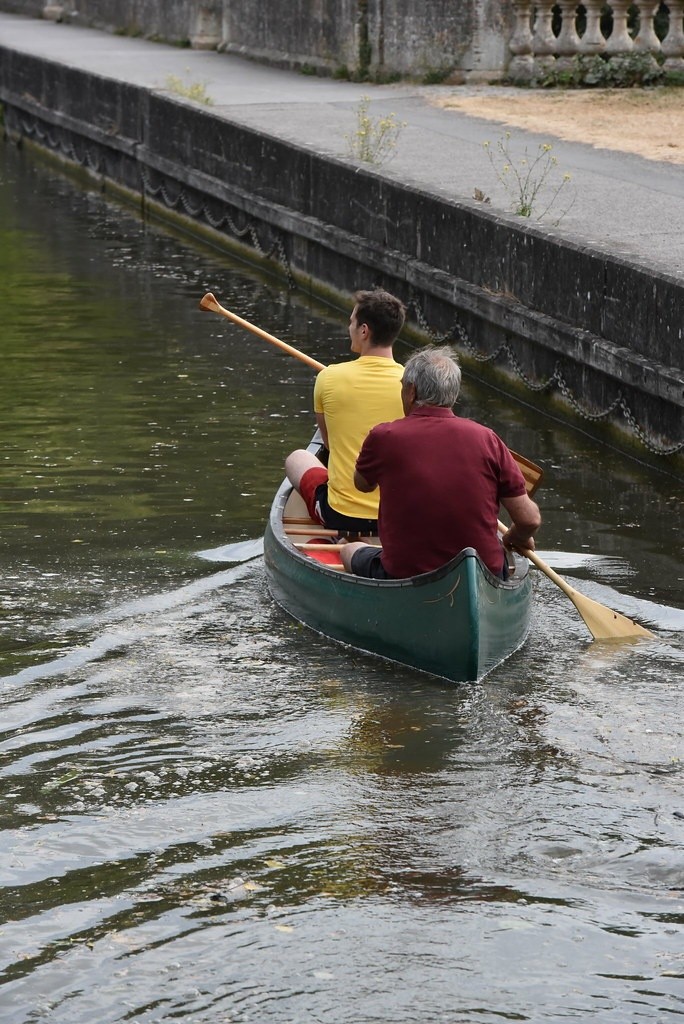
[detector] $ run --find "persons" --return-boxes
[340,343,541,580]
[284,288,408,538]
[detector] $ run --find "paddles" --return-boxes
[198,291,545,500]
[497,517,658,639]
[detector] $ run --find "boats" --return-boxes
[264,426,533,687]
